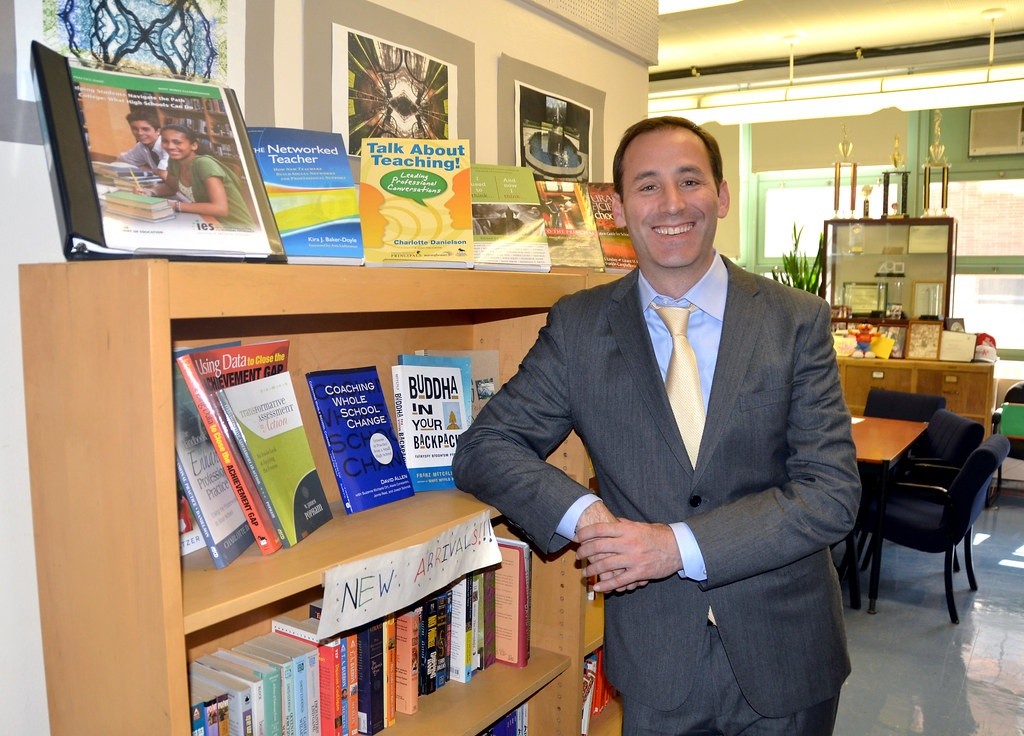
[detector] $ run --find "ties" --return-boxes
[649,302,706,470]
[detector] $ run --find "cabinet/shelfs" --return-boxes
[835,356,993,449]
[20,260,631,736]
[823,217,955,328]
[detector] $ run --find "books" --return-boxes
[469,164,552,273]
[244,126,366,266]
[305,365,415,514]
[103,189,177,223]
[90,159,164,189]
[162,338,333,570]
[580,648,618,736]
[187,537,535,735]
[584,181,639,274]
[390,349,500,494]
[359,135,474,270]
[535,180,606,273]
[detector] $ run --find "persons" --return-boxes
[116,108,254,232]
[836,305,847,318]
[847,322,886,356]
[887,303,900,318]
[451,116,862,736]
[341,689,348,699]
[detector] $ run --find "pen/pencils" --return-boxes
[130,169,142,190]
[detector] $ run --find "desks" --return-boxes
[844,412,926,613]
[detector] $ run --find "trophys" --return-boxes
[879,134,911,219]
[831,120,860,222]
[921,110,953,218]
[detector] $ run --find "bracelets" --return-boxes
[176,200,182,212]
[145,188,158,197]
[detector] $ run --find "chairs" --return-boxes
[835,385,1010,624]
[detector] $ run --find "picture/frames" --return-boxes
[876,322,909,359]
[905,319,945,360]
